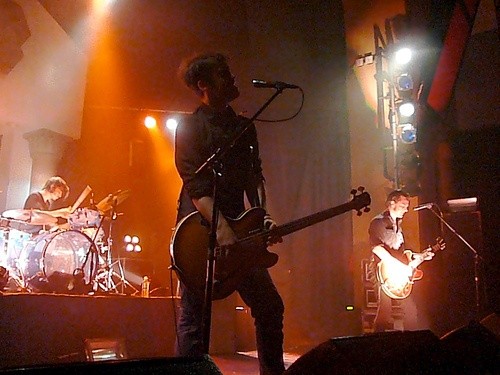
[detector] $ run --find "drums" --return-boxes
[17,229,99,294]
[1,226,32,273]
[70,207,105,241]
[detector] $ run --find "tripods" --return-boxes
[96,217,141,296]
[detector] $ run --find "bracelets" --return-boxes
[264,214,271,219]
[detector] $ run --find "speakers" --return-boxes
[1,354,224,375]
[277,313,500,374]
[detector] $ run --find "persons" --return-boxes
[24,176,71,229]
[368,190,435,333]
[174,48,286,375]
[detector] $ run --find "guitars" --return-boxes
[169,184,373,300]
[376,237,446,300]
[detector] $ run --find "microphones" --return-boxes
[414,203,433,211]
[252,79,300,89]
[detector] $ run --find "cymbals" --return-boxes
[2,209,57,224]
[96,189,130,211]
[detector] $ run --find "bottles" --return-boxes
[142,276,150,298]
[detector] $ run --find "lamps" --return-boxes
[396,72,413,98]
[399,122,417,144]
